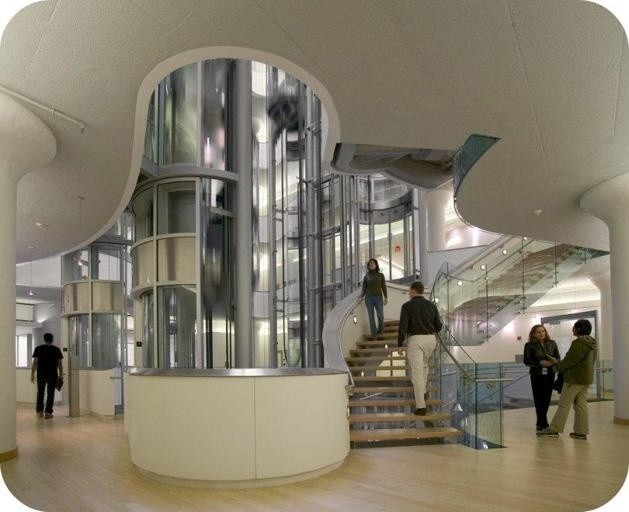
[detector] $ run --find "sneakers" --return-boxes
[538,428,559,437]
[536,426,543,434]
[38,412,43,417]
[45,412,53,419]
[570,432,586,440]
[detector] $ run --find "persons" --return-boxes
[536,319,598,441]
[358,258,388,342]
[30,333,64,420]
[397,281,443,417]
[523,324,561,435]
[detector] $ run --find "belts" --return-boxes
[409,332,433,336]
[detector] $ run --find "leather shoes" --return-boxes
[379,330,384,334]
[414,409,427,416]
[372,336,378,341]
